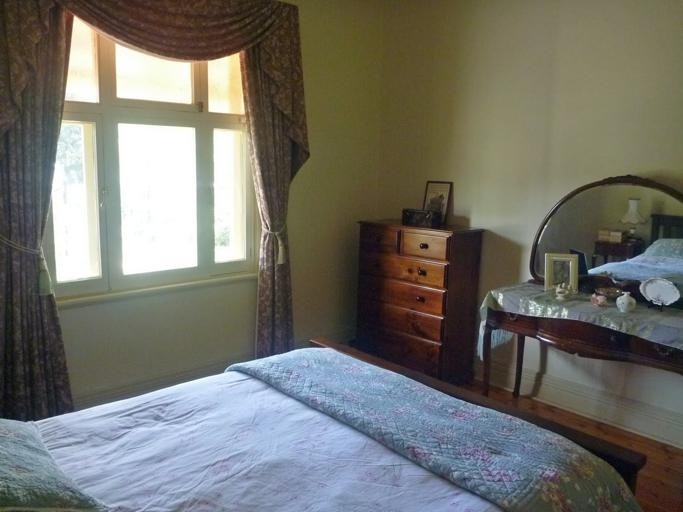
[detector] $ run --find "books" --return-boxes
[597,229,622,243]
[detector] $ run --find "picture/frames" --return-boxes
[543,252,580,295]
[421,178,453,227]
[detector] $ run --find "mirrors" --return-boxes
[528,173,683,310]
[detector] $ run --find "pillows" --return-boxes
[0,419,107,512]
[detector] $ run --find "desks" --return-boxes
[589,240,643,266]
[481,281,683,416]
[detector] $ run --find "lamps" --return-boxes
[620,194,647,244]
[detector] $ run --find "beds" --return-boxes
[0,333,650,512]
[589,213,683,302]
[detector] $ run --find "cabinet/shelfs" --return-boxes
[357,216,483,383]
